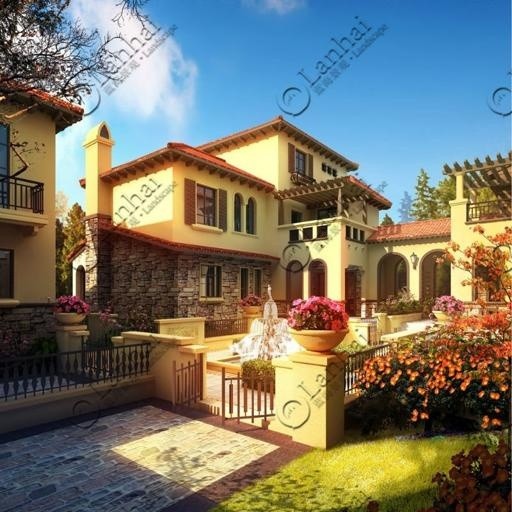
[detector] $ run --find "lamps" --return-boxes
[410,252,417,269]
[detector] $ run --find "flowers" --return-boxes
[287,296,349,330]
[429,295,465,319]
[240,294,264,307]
[52,295,90,314]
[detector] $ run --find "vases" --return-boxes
[54,313,87,324]
[243,306,262,313]
[432,311,453,322]
[288,327,349,352]
